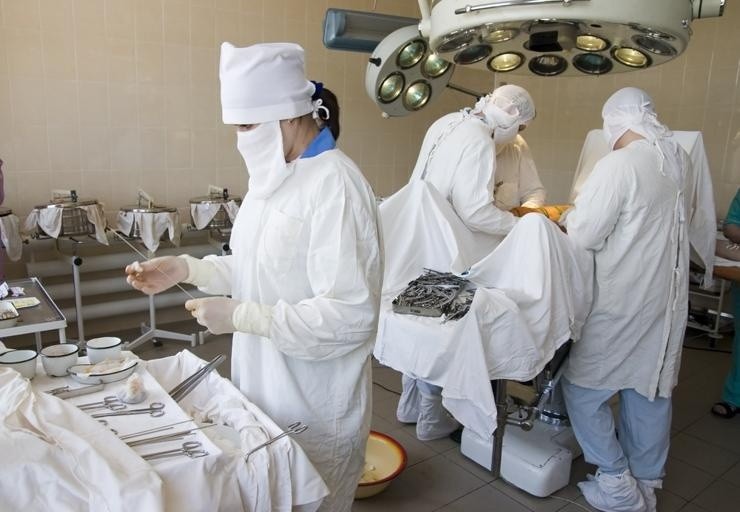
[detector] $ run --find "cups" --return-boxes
[0,348,38,380]
[38,344,80,377]
[84,336,122,365]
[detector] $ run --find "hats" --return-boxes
[600,87,655,120]
[218,41,330,124]
[492,85,536,123]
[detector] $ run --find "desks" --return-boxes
[0,277,67,352]
[0,350,293,510]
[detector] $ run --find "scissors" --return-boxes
[92,403,165,418]
[76,396,125,412]
[244,422,308,461]
[140,441,208,461]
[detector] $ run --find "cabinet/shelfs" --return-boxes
[686,249,724,333]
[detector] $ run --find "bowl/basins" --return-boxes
[354,430,408,500]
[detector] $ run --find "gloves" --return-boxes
[184,297,273,335]
[125,253,213,296]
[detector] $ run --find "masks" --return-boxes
[602,121,629,150]
[494,124,519,143]
[236,121,292,197]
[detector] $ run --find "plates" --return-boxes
[65,363,139,385]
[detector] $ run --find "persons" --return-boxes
[562,85,694,512]
[125,41,385,510]
[710,185,739,419]
[396,83,543,442]
[494,134,546,211]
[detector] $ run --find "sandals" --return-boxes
[711,402,739,418]
